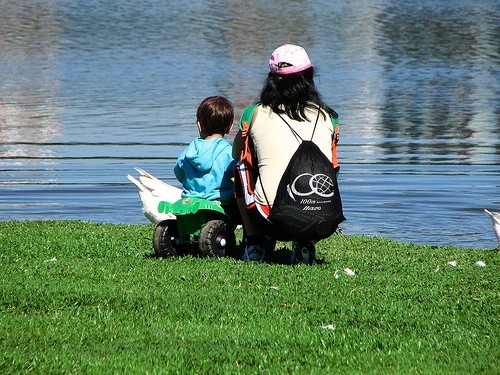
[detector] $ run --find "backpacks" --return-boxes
[258,104,347,241]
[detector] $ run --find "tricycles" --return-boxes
[152,192,277,259]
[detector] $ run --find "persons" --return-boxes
[172,96,246,256]
[233,44,347,262]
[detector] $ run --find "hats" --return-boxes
[269,44,312,74]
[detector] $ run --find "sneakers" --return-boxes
[241,245,265,262]
[291,241,316,266]
